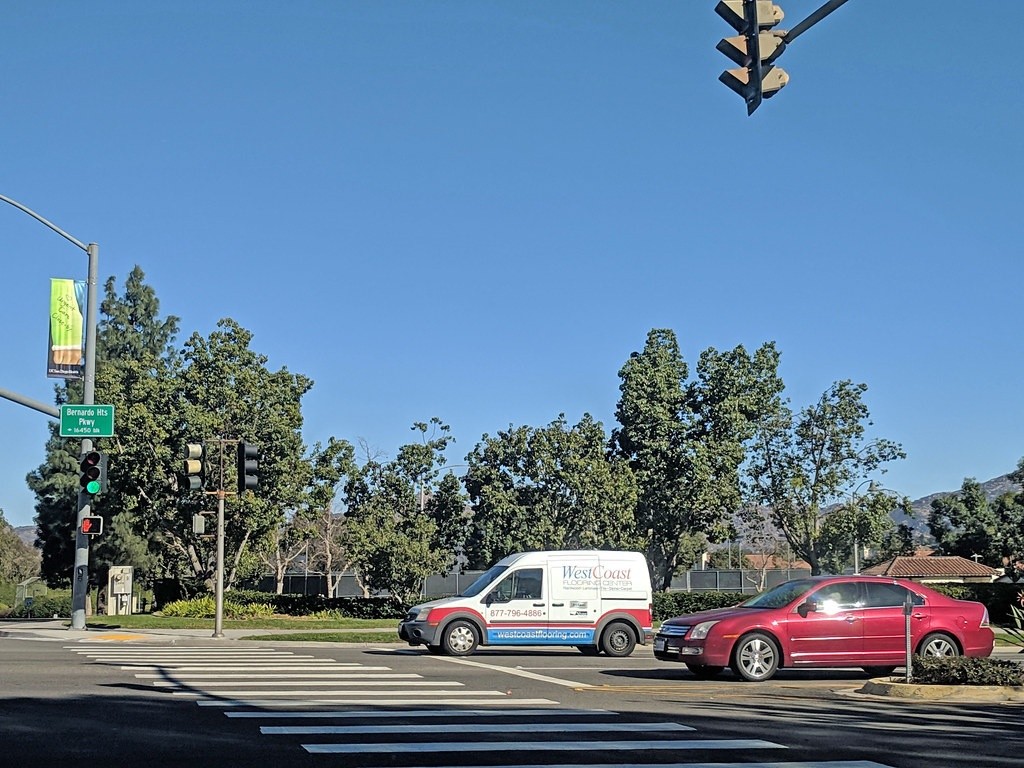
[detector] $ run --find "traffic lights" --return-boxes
[238,443,259,494]
[711,0,789,114]
[182,443,207,491]
[81,451,102,496]
[82,516,104,535]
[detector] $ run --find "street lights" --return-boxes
[852,479,880,574]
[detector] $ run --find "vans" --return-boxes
[397,545,653,657]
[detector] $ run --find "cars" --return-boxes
[653,575,996,683]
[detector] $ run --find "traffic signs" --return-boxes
[60,404,114,436]
